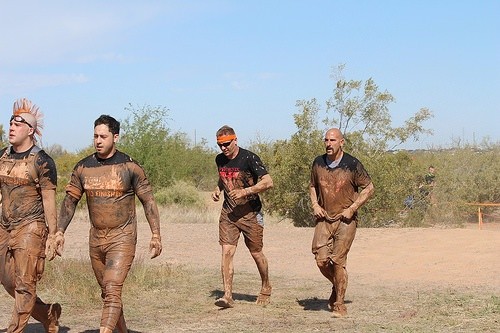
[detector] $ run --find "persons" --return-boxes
[308,128,374,317]
[418,167,440,218]
[56,115,162,333]
[0,98,57,333]
[211,125,275,307]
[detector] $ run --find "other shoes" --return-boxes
[329,286,336,305]
[43,303,61,333]
[214,296,234,308]
[256,284,272,307]
[333,302,349,318]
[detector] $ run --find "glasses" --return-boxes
[217,139,233,147]
[10,115,33,128]
